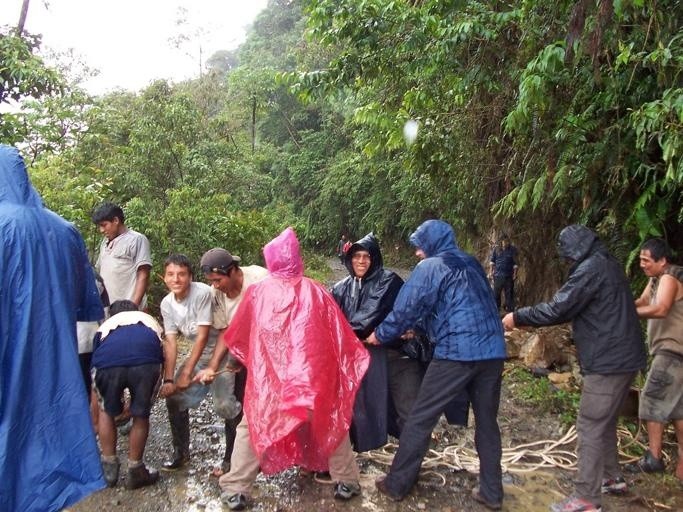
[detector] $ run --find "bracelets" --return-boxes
[163,378,175,384]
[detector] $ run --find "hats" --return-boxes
[200,248,242,274]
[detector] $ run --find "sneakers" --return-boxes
[376,474,403,500]
[125,464,158,490]
[314,471,335,482]
[100,461,119,488]
[601,477,627,494]
[551,495,601,511]
[472,488,503,508]
[213,460,230,476]
[625,451,666,473]
[334,483,361,499]
[220,493,246,510]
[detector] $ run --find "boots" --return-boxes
[162,396,190,471]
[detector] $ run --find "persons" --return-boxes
[217,226,371,512]
[624,237,683,476]
[191,247,322,478]
[0,145,108,511]
[365,218,505,510]
[501,223,648,512]
[90,204,155,434]
[335,235,347,266]
[487,232,519,315]
[314,231,426,485]
[87,299,163,490]
[72,268,109,405]
[159,254,241,474]
